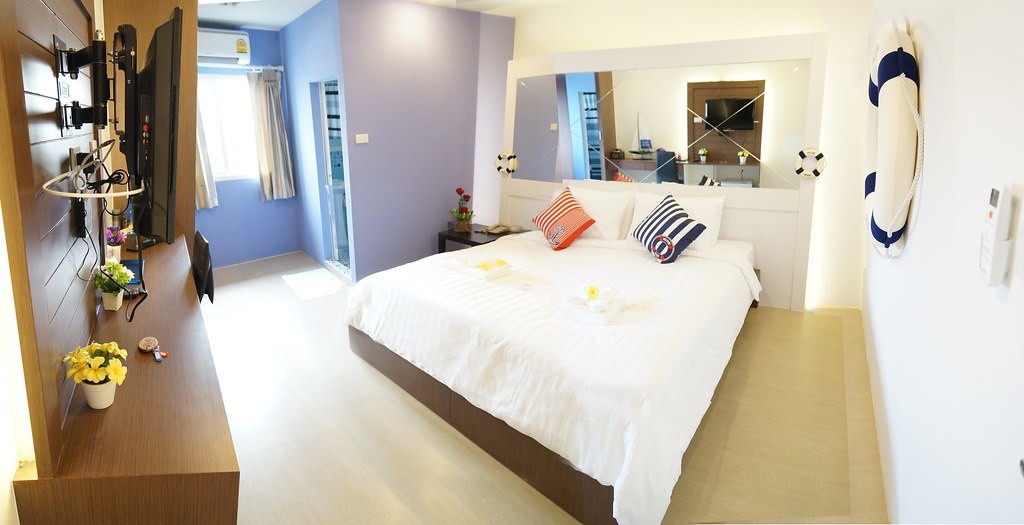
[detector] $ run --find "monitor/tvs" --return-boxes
[705,98,754,131]
[112,5,183,244]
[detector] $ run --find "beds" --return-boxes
[347,230,761,525]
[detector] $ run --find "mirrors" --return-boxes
[510,58,811,190]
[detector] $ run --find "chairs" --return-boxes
[655,151,680,181]
[180,231,218,300]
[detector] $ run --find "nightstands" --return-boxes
[438,222,511,255]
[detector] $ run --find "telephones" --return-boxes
[978,183,1013,287]
[486,224,509,234]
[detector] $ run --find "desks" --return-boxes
[607,159,684,172]
[682,162,761,185]
[54,229,241,524]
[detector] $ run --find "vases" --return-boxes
[454,220,472,232]
[99,288,124,311]
[700,155,706,162]
[81,376,120,409]
[740,156,747,163]
[101,243,121,263]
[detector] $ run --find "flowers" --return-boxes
[738,149,748,157]
[91,261,134,292]
[448,188,476,220]
[698,148,707,155]
[61,340,128,384]
[103,227,130,243]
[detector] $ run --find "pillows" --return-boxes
[625,194,727,246]
[630,192,707,264]
[612,173,633,183]
[698,174,725,186]
[532,190,594,251]
[548,188,638,240]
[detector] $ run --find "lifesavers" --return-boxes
[863,21,919,260]
[495,152,516,174]
[794,149,825,178]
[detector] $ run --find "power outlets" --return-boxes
[740,168,745,173]
[89,140,99,159]
[70,146,82,171]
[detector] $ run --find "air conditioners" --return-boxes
[191,30,251,66]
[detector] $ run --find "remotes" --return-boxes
[122,288,139,299]
